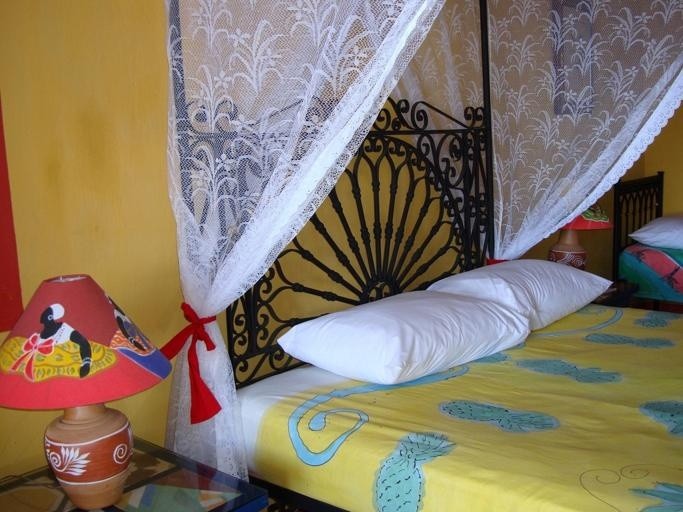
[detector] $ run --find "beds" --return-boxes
[184,102,683,510]
[609,171,683,303]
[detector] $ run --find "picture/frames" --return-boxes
[550,2,594,117]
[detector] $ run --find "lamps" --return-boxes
[0,263,173,508]
[546,178,612,273]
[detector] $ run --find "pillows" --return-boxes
[427,249,613,332]
[628,212,683,250]
[276,250,531,386]
